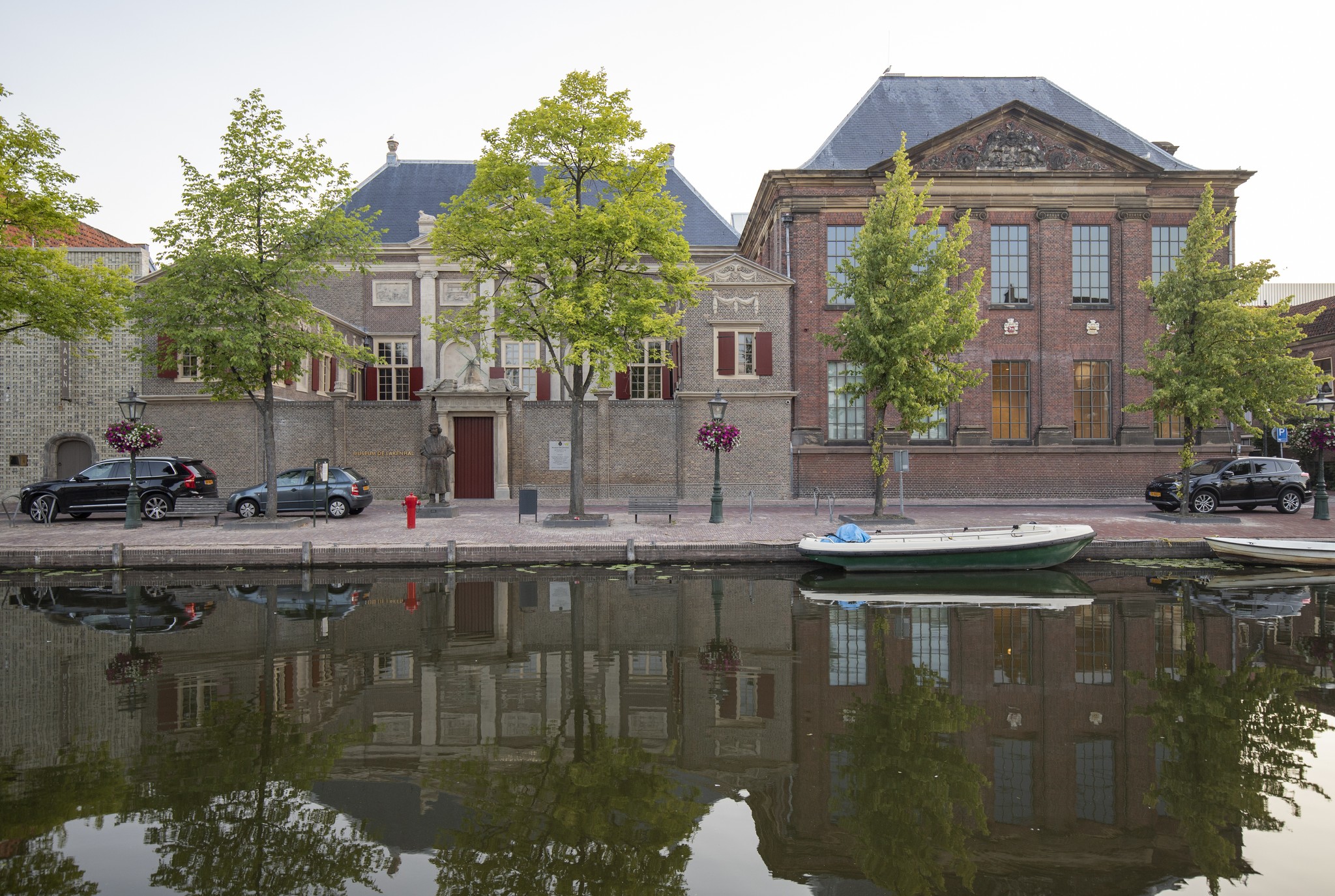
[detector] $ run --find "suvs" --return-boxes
[8,586,220,635]
[1144,457,1313,514]
[19,457,219,522]
[1144,576,1313,620]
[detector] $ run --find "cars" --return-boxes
[225,467,373,522]
[225,582,374,620]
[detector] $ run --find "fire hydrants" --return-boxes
[404,582,420,615]
[401,492,420,528]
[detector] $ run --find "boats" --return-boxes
[796,521,1097,573]
[1205,563,1334,590]
[796,567,1097,612]
[1203,537,1335,567]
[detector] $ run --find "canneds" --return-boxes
[574,517,579,520]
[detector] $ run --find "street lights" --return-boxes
[706,387,728,524]
[1305,388,1335,520]
[1257,405,1274,457]
[116,386,149,530]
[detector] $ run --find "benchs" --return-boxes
[163,496,229,527]
[627,495,680,523]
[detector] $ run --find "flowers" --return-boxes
[103,421,164,457]
[694,421,743,453]
[697,638,744,676]
